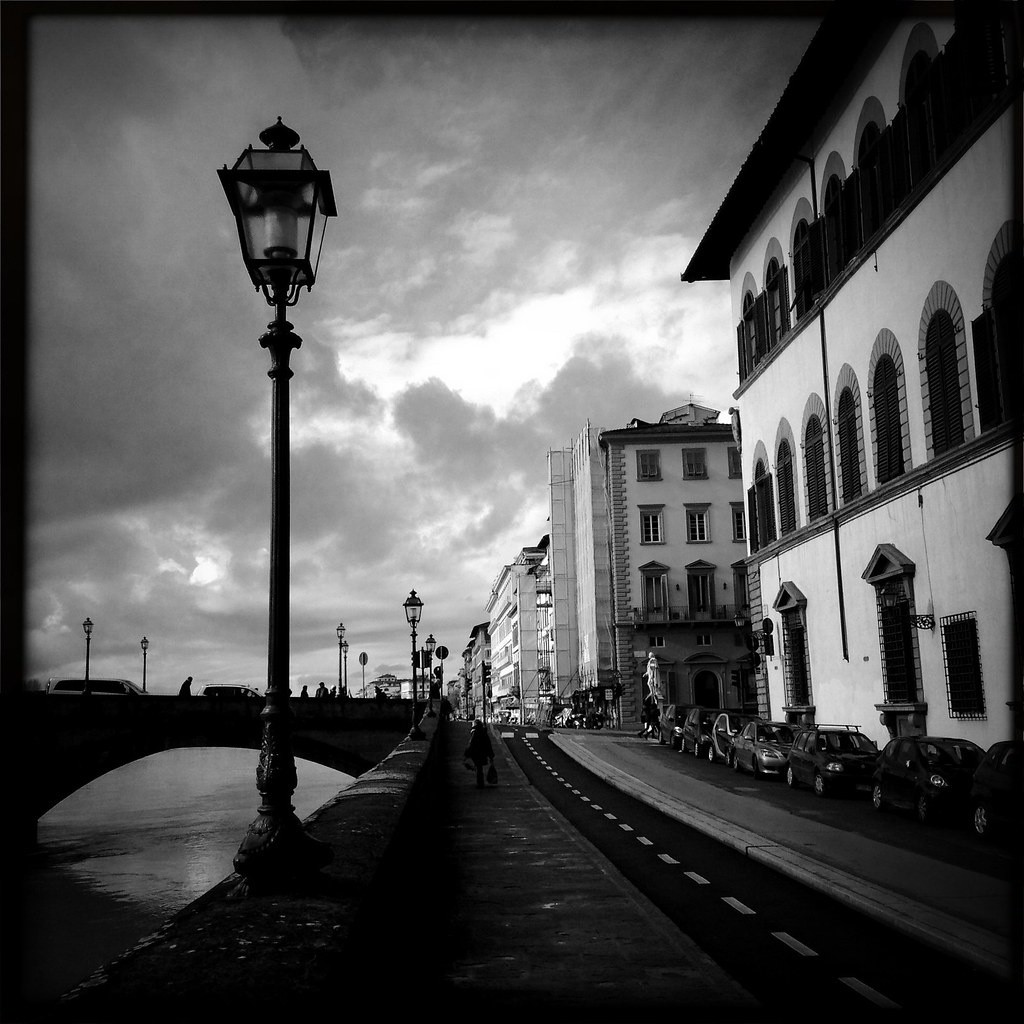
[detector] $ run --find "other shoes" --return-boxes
[476,786,484,789]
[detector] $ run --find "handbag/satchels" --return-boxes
[486,765,498,784]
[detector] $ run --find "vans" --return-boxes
[46,674,151,697]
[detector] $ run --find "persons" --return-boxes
[637,700,658,738]
[300,685,308,697]
[315,682,328,698]
[463,719,495,789]
[179,676,193,695]
[375,686,387,699]
[642,704,661,740]
[643,651,664,701]
[427,674,440,699]
[329,685,337,697]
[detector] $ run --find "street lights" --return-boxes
[81,618,93,698]
[216,116,336,870]
[139,637,150,693]
[426,634,437,718]
[402,590,426,740]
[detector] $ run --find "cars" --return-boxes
[873,735,987,821]
[708,712,762,767]
[969,739,1012,841]
[196,684,266,697]
[658,704,699,747]
[786,724,882,797]
[682,707,737,759]
[733,719,795,777]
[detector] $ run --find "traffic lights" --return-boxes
[464,675,472,693]
[481,663,491,683]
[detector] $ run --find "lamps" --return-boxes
[878,583,935,629]
[733,610,765,640]
